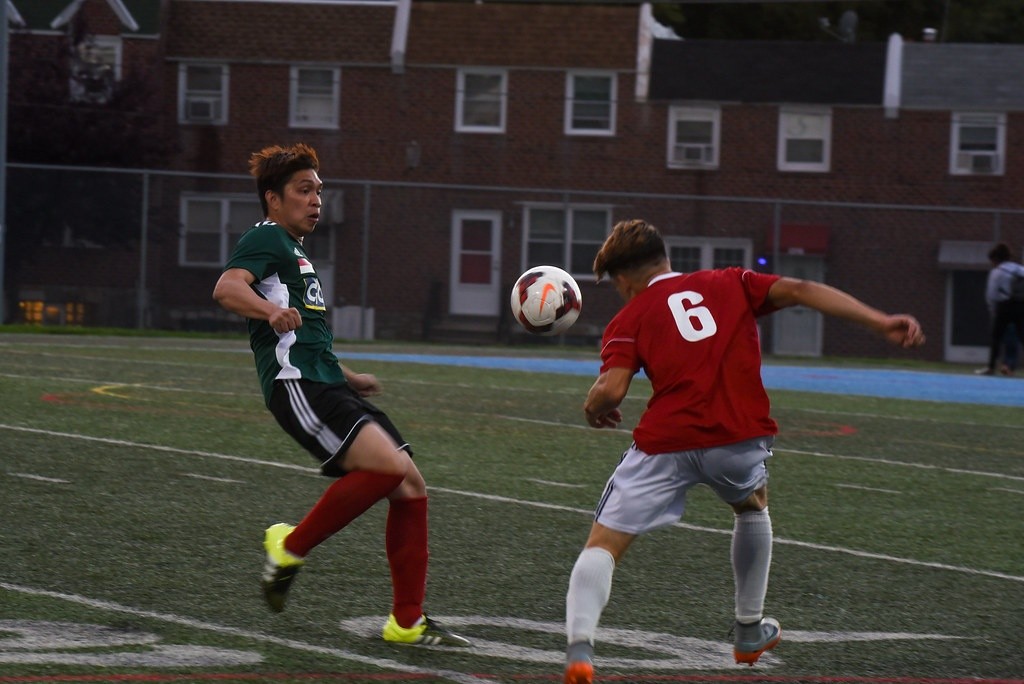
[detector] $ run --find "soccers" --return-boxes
[510,265,583,335]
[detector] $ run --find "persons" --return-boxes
[564,218,924,684]
[212,142,472,648]
[975,244,1024,375]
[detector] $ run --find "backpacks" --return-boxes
[996,267,1024,314]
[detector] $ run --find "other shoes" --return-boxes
[998,364,1015,377]
[975,367,994,375]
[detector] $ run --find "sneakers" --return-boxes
[382,612,471,649]
[566,641,595,684]
[261,523,305,611]
[735,617,781,666]
